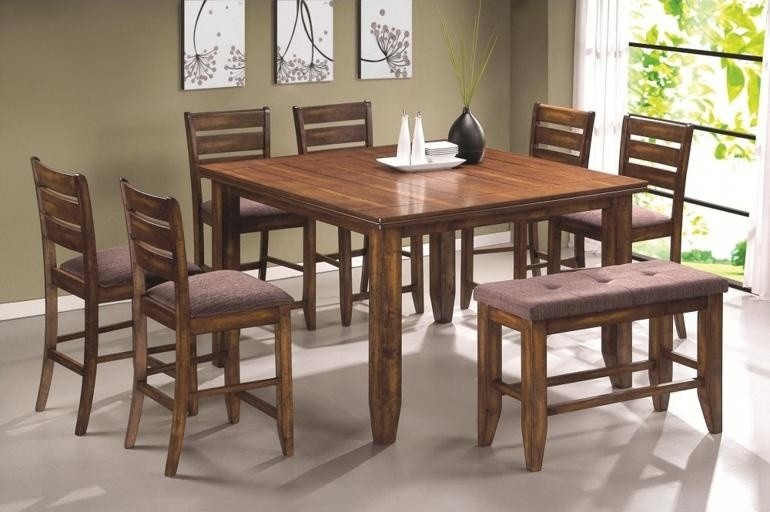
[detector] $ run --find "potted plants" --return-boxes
[438,1,499,169]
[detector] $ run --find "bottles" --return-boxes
[411,112,425,164]
[396,113,410,165]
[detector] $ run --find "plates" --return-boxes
[376,155,465,172]
[425,141,459,161]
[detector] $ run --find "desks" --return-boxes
[193,136,651,443]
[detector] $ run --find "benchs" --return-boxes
[473,257,736,472]
